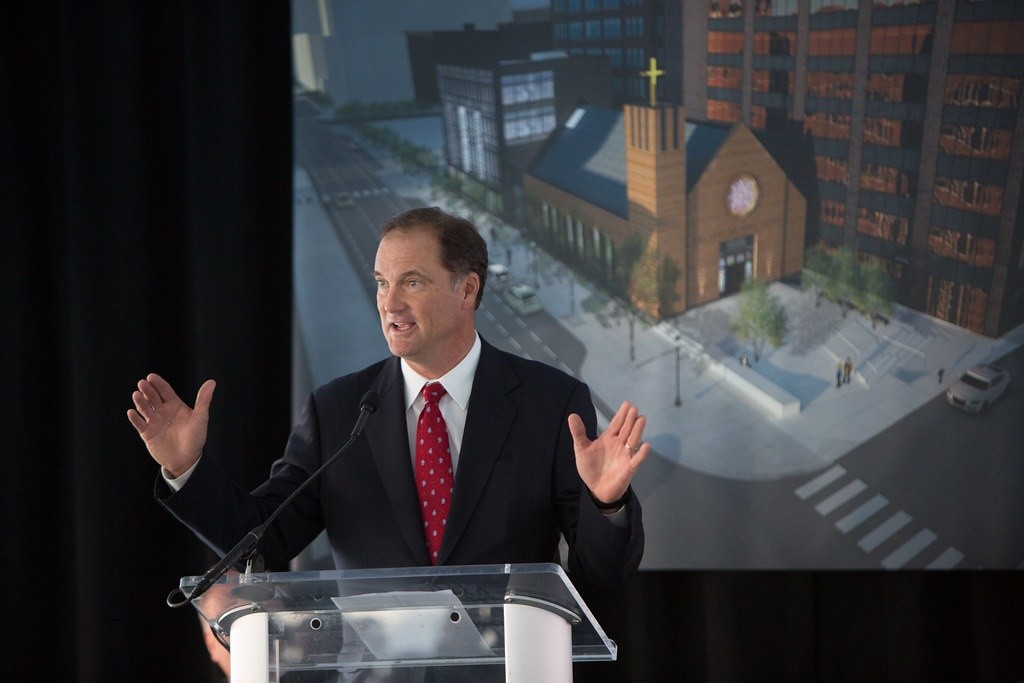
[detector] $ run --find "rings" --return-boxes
[625,442,638,451]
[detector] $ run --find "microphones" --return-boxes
[349,391,381,437]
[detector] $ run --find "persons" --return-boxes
[127,207,652,683]
[836,360,853,388]
[939,369,945,383]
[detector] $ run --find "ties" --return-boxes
[417,382,455,563]
